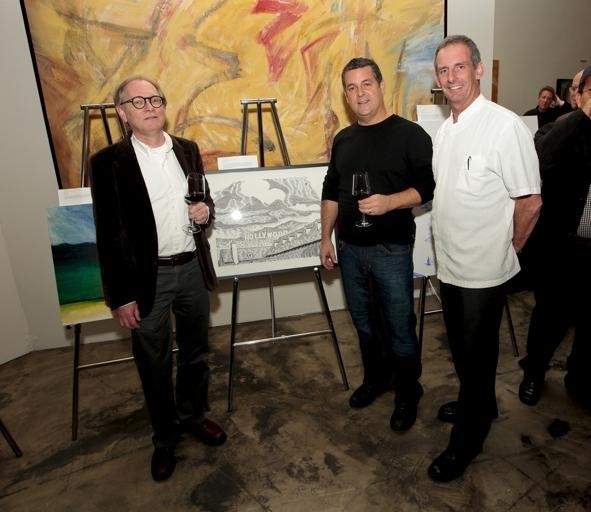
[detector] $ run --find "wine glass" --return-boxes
[352,170,374,229]
[181,171,207,236]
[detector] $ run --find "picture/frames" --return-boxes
[21,0,448,190]
[202,162,339,280]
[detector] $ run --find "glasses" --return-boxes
[118,95,166,110]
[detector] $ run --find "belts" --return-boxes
[156,251,200,268]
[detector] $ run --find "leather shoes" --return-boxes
[429,444,482,483]
[438,401,500,423]
[390,380,423,431]
[177,414,228,446]
[150,430,179,483]
[349,381,393,409]
[519,370,546,406]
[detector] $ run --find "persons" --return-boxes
[318,56,435,434]
[91,77,228,482]
[517,66,591,408]
[424,34,544,484]
